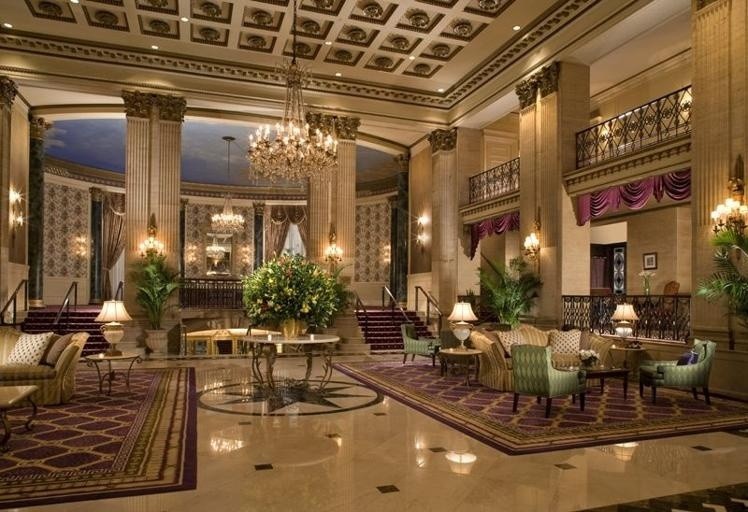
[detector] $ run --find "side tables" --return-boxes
[440,349,485,390]
[84,353,141,395]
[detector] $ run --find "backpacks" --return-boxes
[676,350,698,366]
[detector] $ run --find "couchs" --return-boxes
[473,327,594,392]
[0,325,90,407]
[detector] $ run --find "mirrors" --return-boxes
[205,233,233,277]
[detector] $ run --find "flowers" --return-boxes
[578,349,600,361]
[241,252,342,328]
[637,270,657,280]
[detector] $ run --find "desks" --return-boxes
[242,335,343,405]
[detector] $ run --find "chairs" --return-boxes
[510,342,588,418]
[638,339,717,405]
[400,323,439,368]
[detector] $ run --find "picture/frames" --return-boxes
[643,252,657,270]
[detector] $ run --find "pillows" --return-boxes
[7,330,54,365]
[497,330,526,357]
[45,333,73,365]
[551,328,582,353]
[677,352,698,365]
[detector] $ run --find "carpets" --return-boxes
[332,351,748,457]
[0,366,197,509]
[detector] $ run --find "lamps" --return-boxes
[206,237,226,268]
[448,298,479,352]
[710,197,746,240]
[141,239,166,257]
[524,232,541,262]
[94,301,134,357]
[609,305,640,347]
[246,0,338,183]
[207,134,246,235]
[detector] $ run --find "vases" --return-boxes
[585,359,596,368]
[280,317,302,338]
[644,280,651,297]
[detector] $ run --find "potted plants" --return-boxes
[129,249,185,353]
